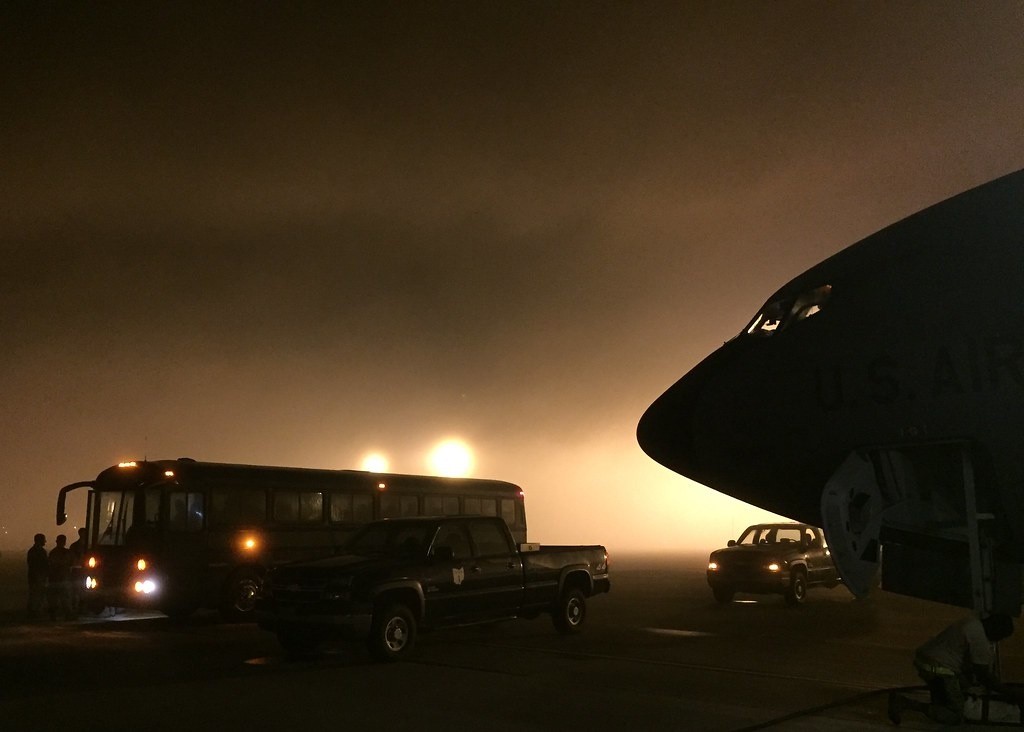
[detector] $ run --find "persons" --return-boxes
[26,528,87,619]
[888,614,1014,727]
[174,498,187,524]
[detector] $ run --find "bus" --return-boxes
[54,457,528,620]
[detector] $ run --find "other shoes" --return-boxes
[888,691,904,725]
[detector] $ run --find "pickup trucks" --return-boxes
[706,521,844,606]
[242,514,612,660]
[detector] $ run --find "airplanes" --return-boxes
[638,167,1024,623]
